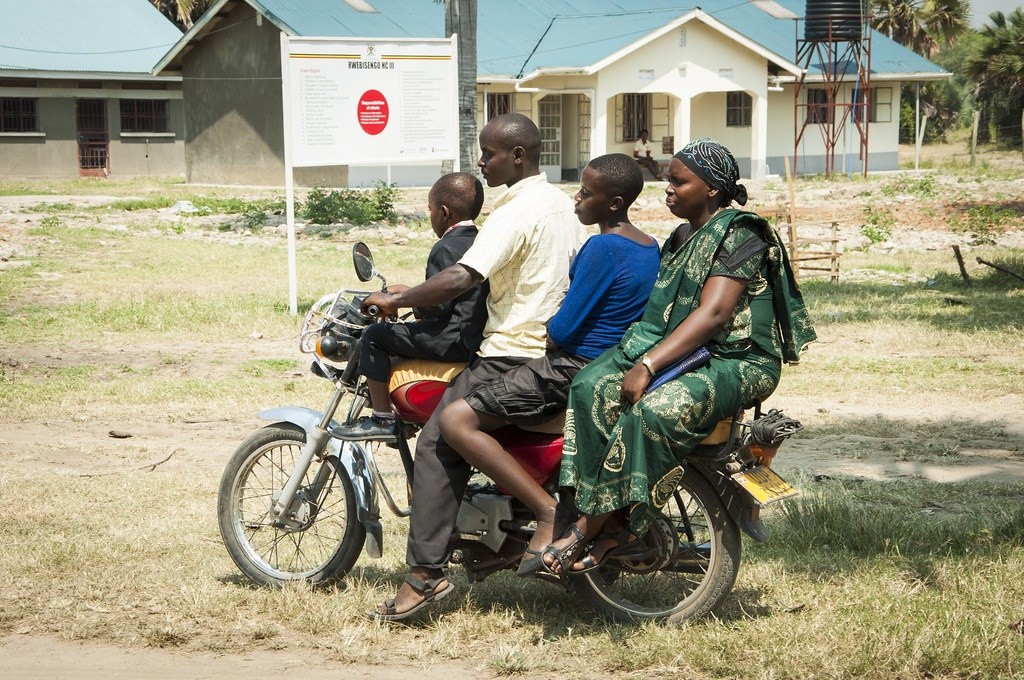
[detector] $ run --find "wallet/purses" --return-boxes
[643,345,711,395]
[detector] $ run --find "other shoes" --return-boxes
[517,507,573,577]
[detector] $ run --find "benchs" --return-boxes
[640,162,668,174]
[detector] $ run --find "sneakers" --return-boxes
[330,416,400,443]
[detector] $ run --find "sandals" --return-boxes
[568,527,641,575]
[368,568,454,620]
[540,513,602,576]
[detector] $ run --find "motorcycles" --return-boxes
[218,242,804,629]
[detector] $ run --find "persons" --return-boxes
[436,155,661,577]
[540,137,819,581]
[327,172,490,440]
[631,128,668,182]
[358,113,592,622]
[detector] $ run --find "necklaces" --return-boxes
[682,227,692,245]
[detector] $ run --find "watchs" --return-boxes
[641,353,656,377]
